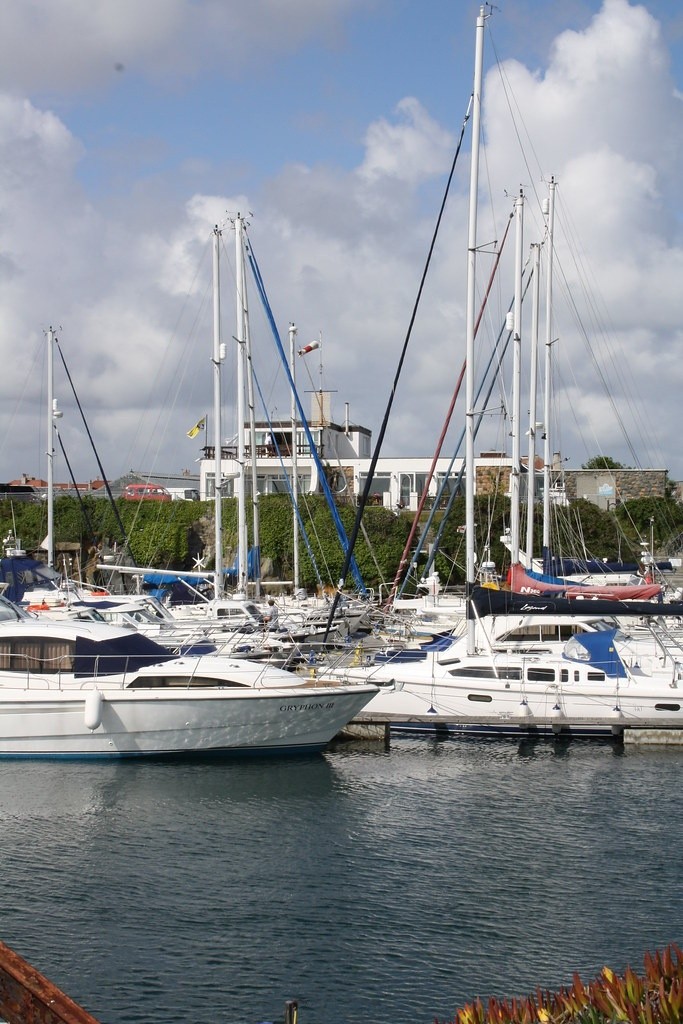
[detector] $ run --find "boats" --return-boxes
[0,617,381,762]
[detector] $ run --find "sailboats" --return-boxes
[295,1,683,744]
[0,211,370,671]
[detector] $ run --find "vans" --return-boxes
[122,484,171,502]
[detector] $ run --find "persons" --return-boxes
[645,566,655,585]
[262,600,279,633]
[294,583,307,609]
[41,599,50,610]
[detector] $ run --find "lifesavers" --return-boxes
[91,592,109,596]
[27,605,50,610]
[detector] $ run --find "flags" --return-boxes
[187,417,206,439]
